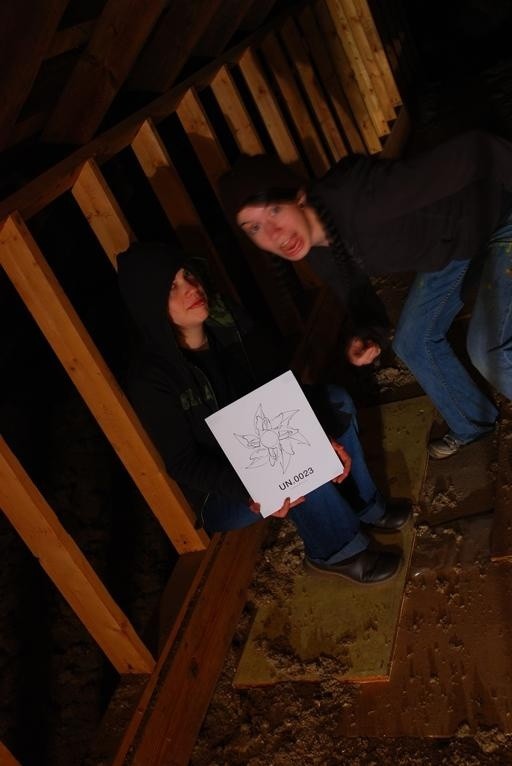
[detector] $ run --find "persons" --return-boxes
[115,246,414,582]
[221,128,512,460]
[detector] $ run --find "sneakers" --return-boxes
[357,508,413,531]
[304,542,401,586]
[429,432,473,460]
